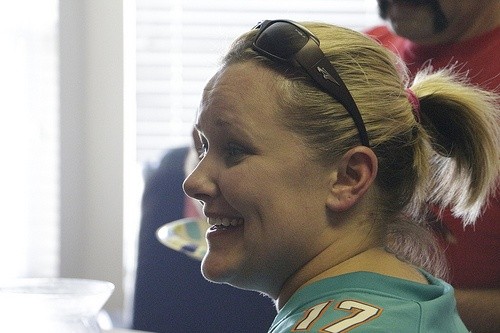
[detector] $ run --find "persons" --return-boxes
[359,0,500,333]
[133,145,278,333]
[183,19,500,333]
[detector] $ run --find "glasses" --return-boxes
[252,18,371,148]
